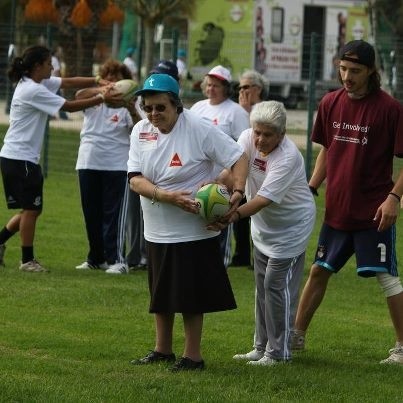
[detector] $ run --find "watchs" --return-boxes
[233,189,245,198]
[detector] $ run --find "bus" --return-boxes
[185,0,370,110]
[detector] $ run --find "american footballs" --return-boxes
[110,79,140,103]
[195,182,233,222]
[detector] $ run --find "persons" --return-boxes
[0,46,270,276]
[126,74,247,373]
[291,40,403,365]
[198,101,315,366]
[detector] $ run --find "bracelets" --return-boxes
[388,192,400,201]
[309,185,318,195]
[151,185,159,205]
[97,93,105,104]
[236,211,240,220]
[94,75,101,84]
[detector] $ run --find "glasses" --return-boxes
[238,84,253,91]
[143,104,171,112]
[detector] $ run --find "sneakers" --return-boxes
[19,259,47,272]
[247,355,285,365]
[105,260,130,274]
[75,261,108,270]
[290,330,305,351]
[232,349,265,360]
[379,342,403,364]
[131,349,175,364]
[0,244,6,265]
[171,357,204,371]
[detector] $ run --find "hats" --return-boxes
[205,65,231,84]
[340,39,375,68]
[149,61,179,82]
[134,74,180,97]
